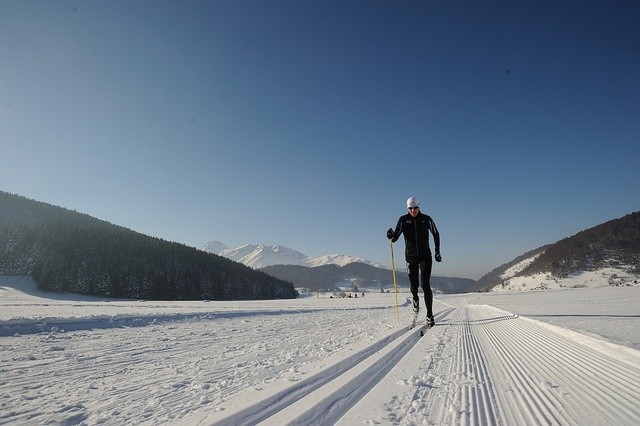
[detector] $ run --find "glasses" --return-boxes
[407,206,419,210]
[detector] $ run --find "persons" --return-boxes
[386,198,442,330]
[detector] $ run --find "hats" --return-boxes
[407,197,420,208]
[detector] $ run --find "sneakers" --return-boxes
[426,315,435,327]
[413,297,419,311]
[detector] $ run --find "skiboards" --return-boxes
[409,323,431,336]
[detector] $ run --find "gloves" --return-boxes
[387,228,394,239]
[435,254,442,262]
